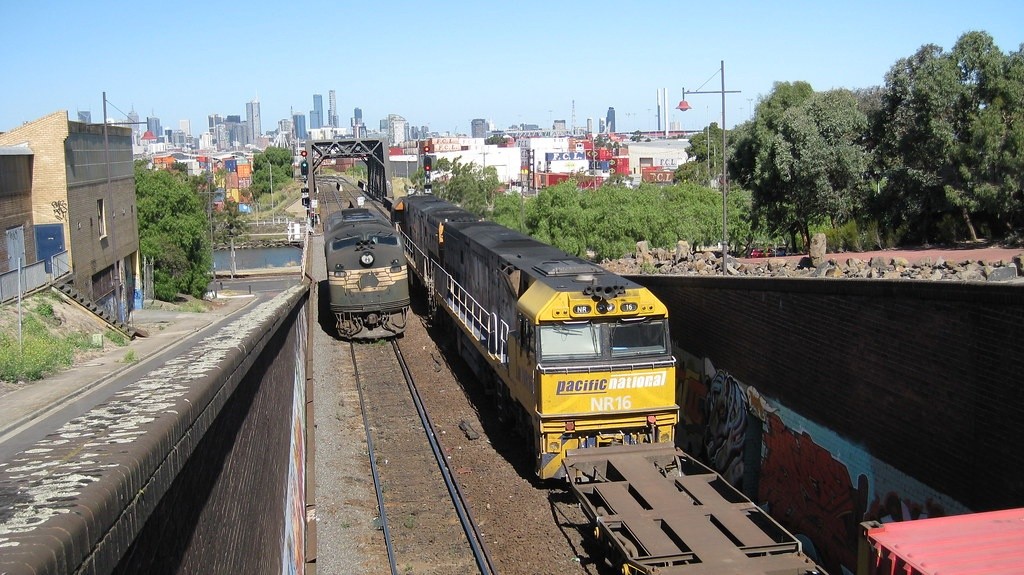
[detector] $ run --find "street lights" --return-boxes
[103,92,157,324]
[674,86,727,276]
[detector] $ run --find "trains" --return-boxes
[322,207,409,340]
[389,195,681,481]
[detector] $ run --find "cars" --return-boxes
[750,246,786,258]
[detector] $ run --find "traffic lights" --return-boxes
[424,157,432,171]
[301,161,309,175]
